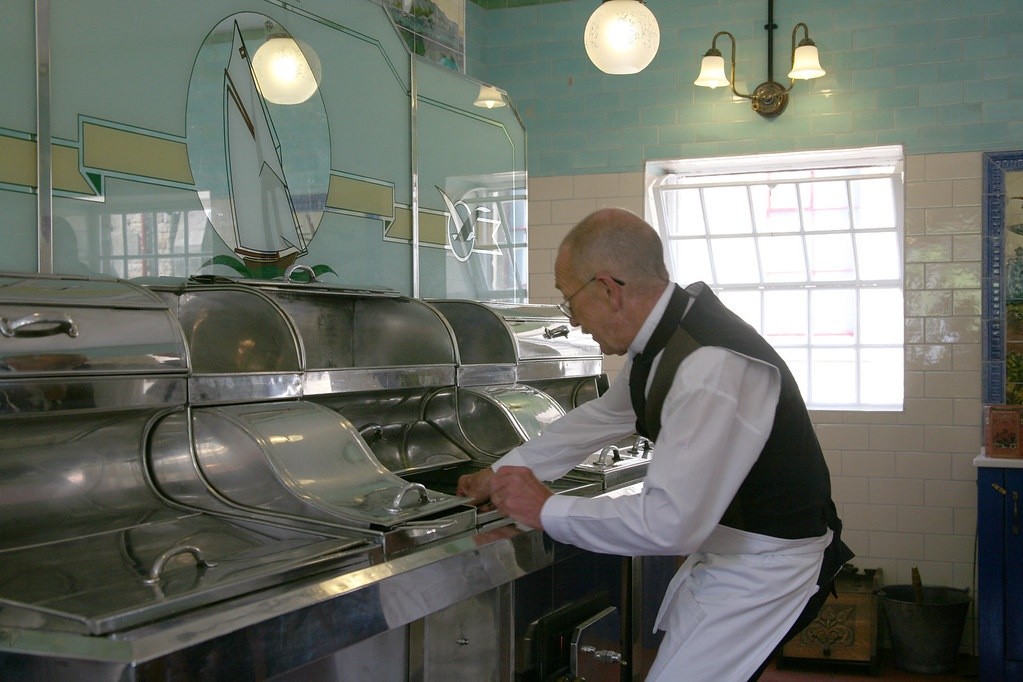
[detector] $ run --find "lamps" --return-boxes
[248,19,322,105]
[472,85,508,110]
[695,0,826,120]
[584,0,660,74]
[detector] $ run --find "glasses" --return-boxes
[556,276,626,319]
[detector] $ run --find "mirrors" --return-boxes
[185,10,332,262]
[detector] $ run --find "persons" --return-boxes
[456,209,854,682]
[0,354,90,416]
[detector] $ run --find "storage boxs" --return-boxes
[774,575,881,676]
